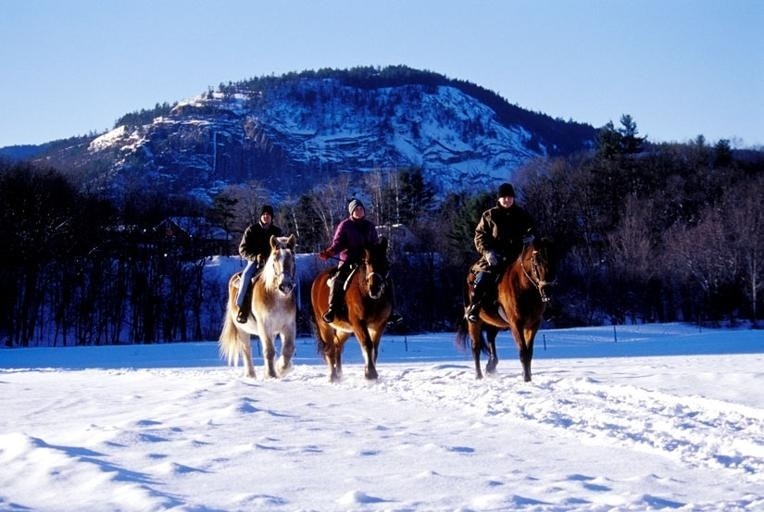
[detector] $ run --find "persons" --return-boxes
[235,204,305,324]
[319,199,404,326]
[468,183,555,325]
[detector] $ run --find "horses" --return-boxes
[310,233,396,380]
[453,230,560,383]
[215,233,301,380]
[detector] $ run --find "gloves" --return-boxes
[486,252,497,267]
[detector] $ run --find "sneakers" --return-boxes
[323,309,335,322]
[469,314,477,323]
[392,314,403,321]
[237,311,247,322]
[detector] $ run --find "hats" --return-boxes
[497,183,515,197]
[260,205,273,218]
[349,199,364,214]
